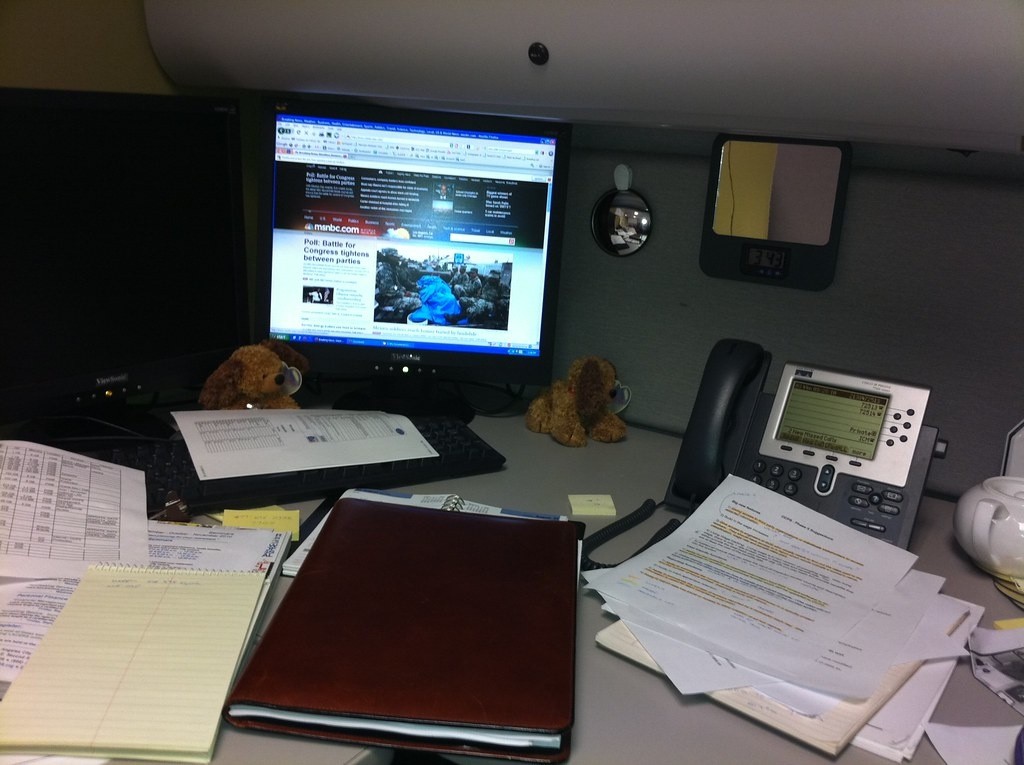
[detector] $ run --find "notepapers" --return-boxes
[993,618,1024,631]
[568,494,617,516]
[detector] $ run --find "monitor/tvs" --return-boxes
[254,96,574,388]
[1,87,250,421]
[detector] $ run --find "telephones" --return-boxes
[664,338,949,551]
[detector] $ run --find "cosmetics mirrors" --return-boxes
[591,188,652,255]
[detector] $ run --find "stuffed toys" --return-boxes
[525,354,627,448]
[199,342,301,411]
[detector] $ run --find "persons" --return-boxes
[448,265,511,324]
[436,184,451,201]
[309,288,322,303]
[376,247,422,322]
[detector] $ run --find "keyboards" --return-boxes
[96,414,507,516]
